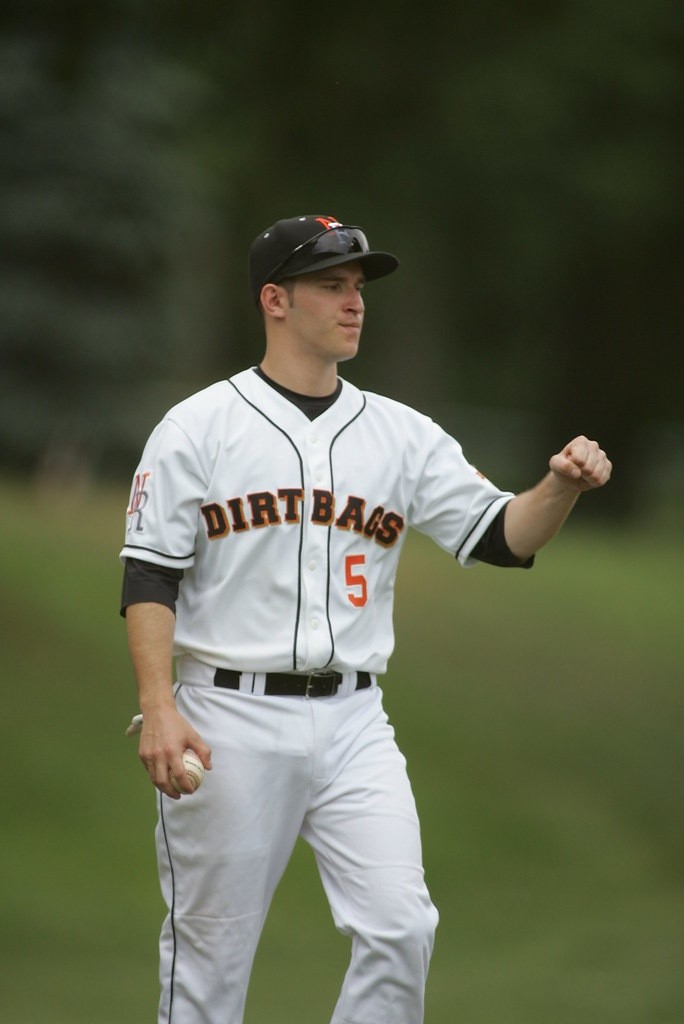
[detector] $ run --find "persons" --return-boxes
[118,216,611,1024]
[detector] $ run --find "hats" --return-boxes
[250,215,399,301]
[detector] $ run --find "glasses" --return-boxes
[265,225,370,280]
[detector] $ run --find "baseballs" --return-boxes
[167,750,205,794]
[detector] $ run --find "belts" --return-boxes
[214,668,371,700]
